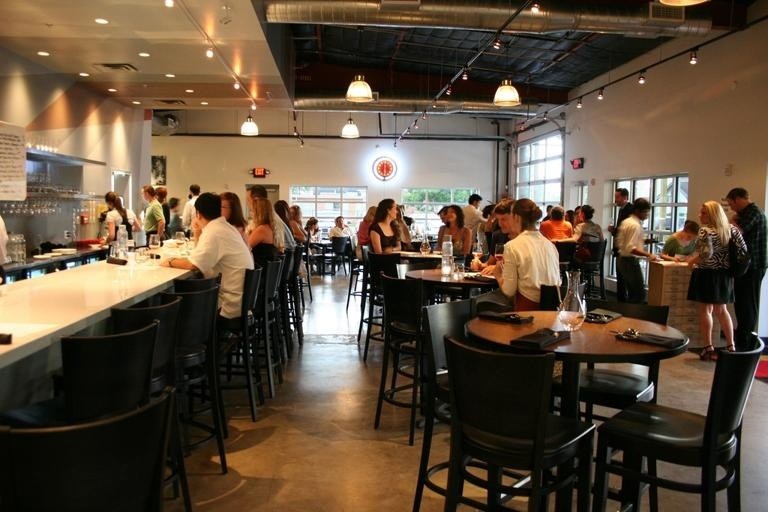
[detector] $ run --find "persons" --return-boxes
[357,189,603,311]
[617,198,656,304]
[220,193,250,250]
[246,185,356,272]
[688,200,747,360]
[660,220,701,262]
[98,185,201,246]
[0,214,10,287]
[609,187,634,302]
[727,187,767,347]
[160,192,254,343]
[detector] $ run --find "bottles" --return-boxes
[7,234,26,262]
[117,224,128,257]
[442,234,453,276]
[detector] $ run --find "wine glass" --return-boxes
[148,234,161,266]
[471,242,483,272]
[175,231,184,257]
[0,199,63,216]
[134,248,147,272]
[494,243,503,270]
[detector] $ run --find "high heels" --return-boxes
[723,344,737,352]
[700,345,715,360]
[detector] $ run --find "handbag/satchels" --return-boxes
[729,237,752,277]
[473,288,515,314]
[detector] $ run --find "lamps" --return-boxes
[346,25,373,103]
[164,0,256,111]
[399,0,534,140]
[494,34,520,106]
[515,14,768,131]
[341,111,360,139]
[241,101,259,136]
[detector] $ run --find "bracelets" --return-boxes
[169,258,175,267]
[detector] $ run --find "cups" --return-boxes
[451,262,465,281]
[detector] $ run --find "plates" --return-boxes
[32,248,77,259]
